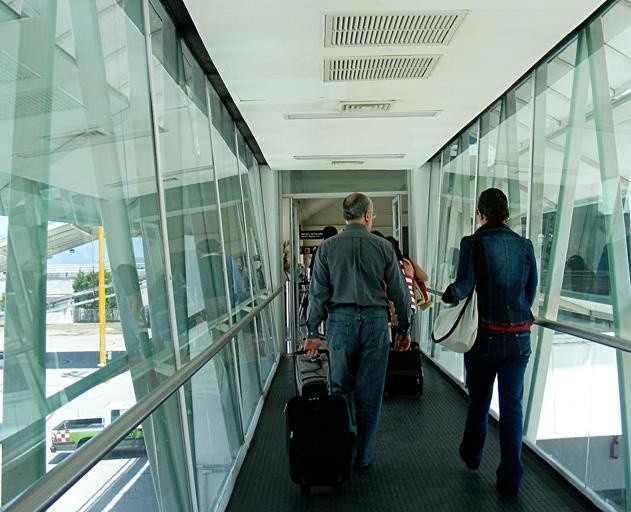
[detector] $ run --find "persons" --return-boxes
[116,237,264,453]
[302,192,412,470]
[308,225,337,279]
[443,185,539,499]
[562,226,631,325]
[371,229,385,238]
[384,236,415,345]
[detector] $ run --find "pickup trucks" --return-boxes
[49,400,146,454]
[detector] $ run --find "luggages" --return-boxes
[294,333,333,396]
[285,349,354,495]
[384,322,425,401]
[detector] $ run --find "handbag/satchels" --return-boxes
[430,290,479,353]
[413,279,433,311]
